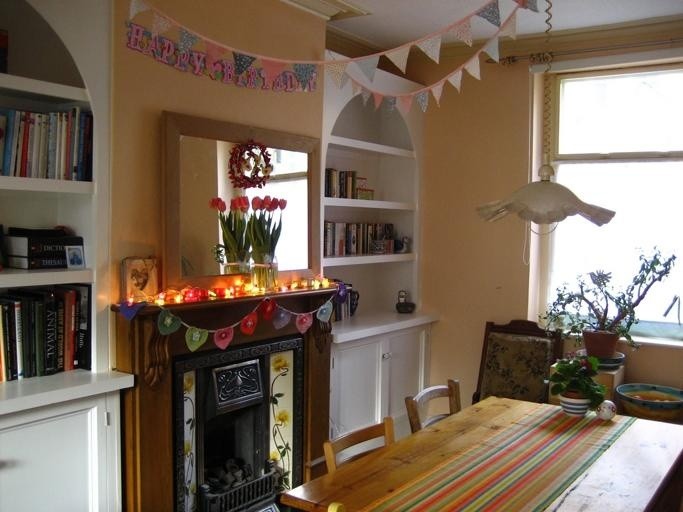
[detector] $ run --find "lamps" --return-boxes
[474,10,616,229]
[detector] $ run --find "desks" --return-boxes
[279,397,683,512]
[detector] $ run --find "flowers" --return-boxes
[207,194,252,274]
[227,138,274,190]
[246,195,286,291]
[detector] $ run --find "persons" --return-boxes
[70,247,82,265]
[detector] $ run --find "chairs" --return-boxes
[323,416,395,473]
[471,319,565,405]
[404,378,462,434]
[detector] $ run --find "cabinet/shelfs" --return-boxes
[0,0,134,512]
[319,49,440,467]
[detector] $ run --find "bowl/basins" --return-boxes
[615,383,682,420]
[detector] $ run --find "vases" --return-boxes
[249,263,278,294]
[224,263,250,275]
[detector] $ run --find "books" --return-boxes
[330,291,352,322]
[2,227,71,269]
[0,285,90,382]
[323,166,359,200]
[1,105,93,182]
[325,220,396,257]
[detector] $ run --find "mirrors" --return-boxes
[160,109,321,292]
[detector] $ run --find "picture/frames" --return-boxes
[64,245,84,269]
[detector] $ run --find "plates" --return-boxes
[577,350,625,367]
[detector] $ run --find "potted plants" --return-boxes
[542,351,608,419]
[536,245,676,360]
